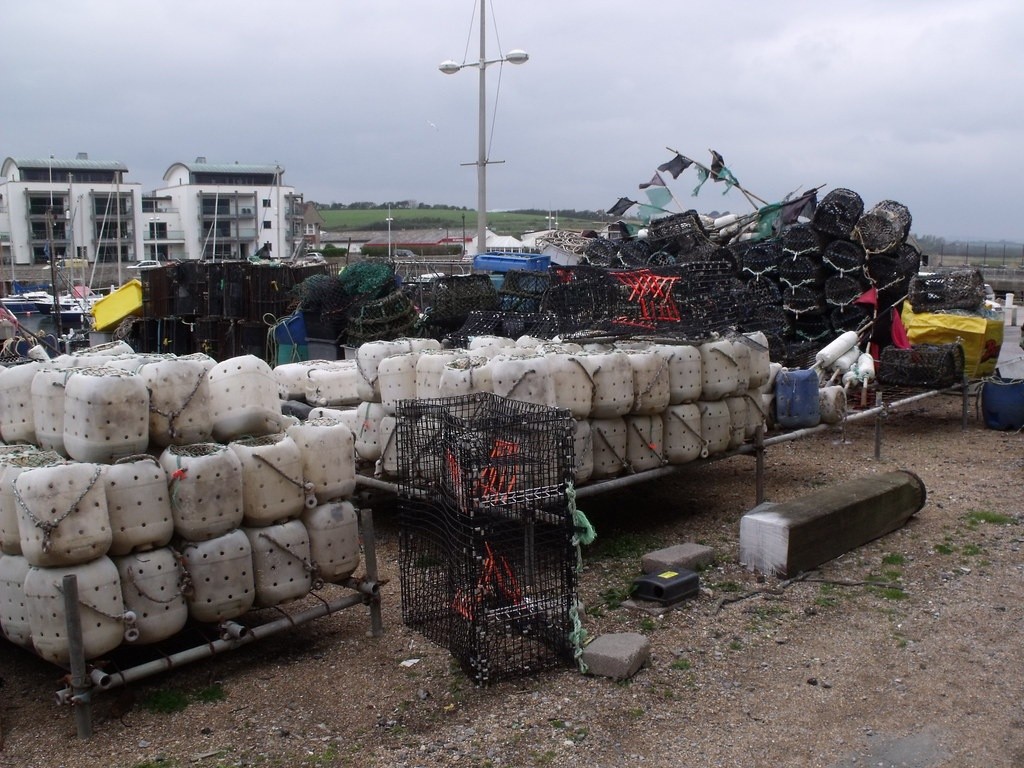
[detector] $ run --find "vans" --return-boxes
[306,252,324,263]
[55,259,88,269]
[393,249,413,257]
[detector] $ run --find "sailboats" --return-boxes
[0,171,120,363]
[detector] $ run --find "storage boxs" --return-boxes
[474,251,552,274]
[541,240,587,267]
[632,565,702,607]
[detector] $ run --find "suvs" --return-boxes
[126,259,162,268]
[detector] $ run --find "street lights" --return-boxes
[439,0,529,273]
[385,204,393,259]
